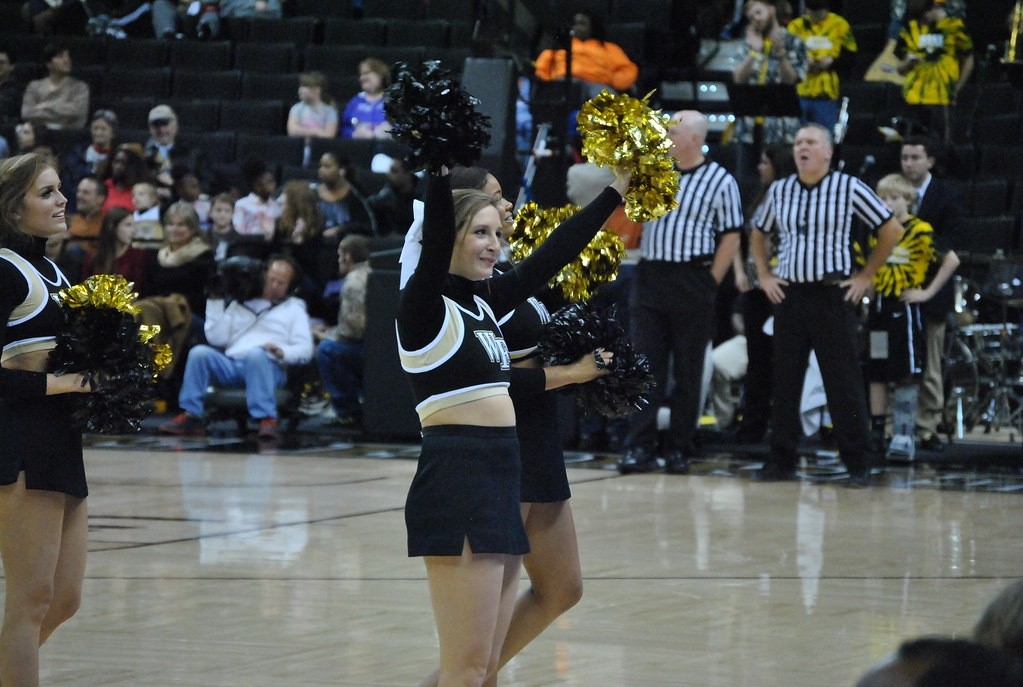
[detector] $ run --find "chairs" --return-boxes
[0,1,1023,337]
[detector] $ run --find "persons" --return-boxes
[0,152,114,686]
[422,164,617,687]
[0,2,980,489]
[393,64,681,686]
[857,580,1022,687]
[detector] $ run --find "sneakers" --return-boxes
[158,411,208,435]
[258,420,280,439]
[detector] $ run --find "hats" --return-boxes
[148,104,174,123]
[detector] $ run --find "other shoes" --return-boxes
[156,27,175,39]
[867,426,941,462]
[197,21,211,40]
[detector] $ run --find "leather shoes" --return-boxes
[746,463,789,482]
[848,472,871,487]
[664,449,689,475]
[616,446,659,473]
